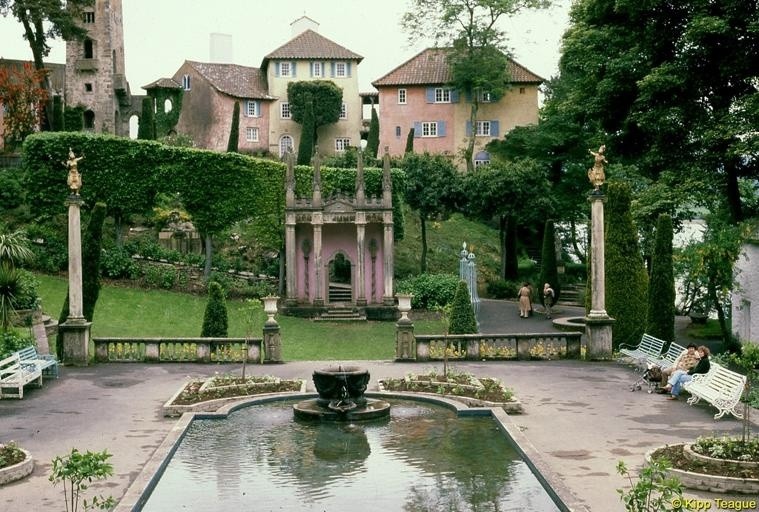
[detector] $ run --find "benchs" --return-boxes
[1,346,58,399]
[619,333,748,421]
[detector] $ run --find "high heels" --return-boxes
[666,397,679,400]
[659,386,672,392]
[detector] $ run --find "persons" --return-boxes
[528,284,534,316]
[60,147,86,195]
[586,143,609,193]
[659,345,710,400]
[517,282,531,319]
[543,283,555,319]
[654,341,699,394]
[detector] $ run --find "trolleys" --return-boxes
[628,364,666,395]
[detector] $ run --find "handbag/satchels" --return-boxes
[649,369,662,382]
